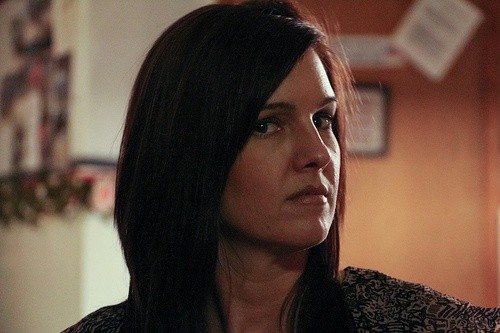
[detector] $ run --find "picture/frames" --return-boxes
[344,80,392,158]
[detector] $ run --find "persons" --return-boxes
[60,2,499,333]
